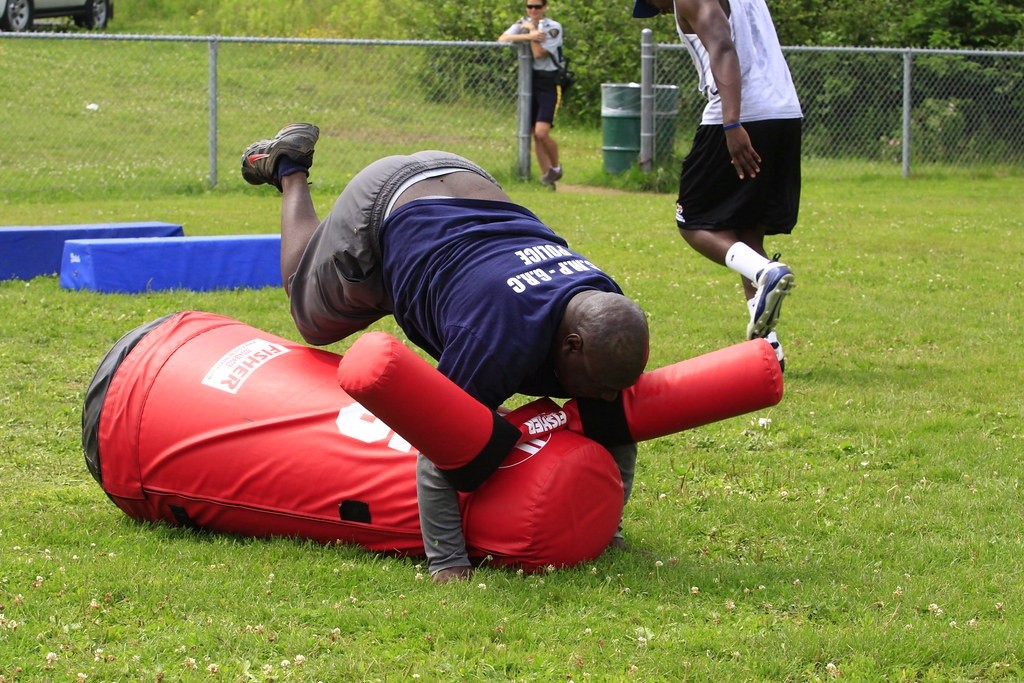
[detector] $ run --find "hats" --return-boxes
[633,0,660,18]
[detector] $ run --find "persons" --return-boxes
[495,0,566,191]
[652,0,803,375]
[239,122,648,586]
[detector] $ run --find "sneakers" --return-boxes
[240,123,319,193]
[747,253,795,375]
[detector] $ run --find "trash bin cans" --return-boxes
[600,82,681,176]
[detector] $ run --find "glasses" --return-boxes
[526,4,544,9]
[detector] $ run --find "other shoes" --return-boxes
[541,164,563,191]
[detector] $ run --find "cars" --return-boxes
[0,0,113,36]
